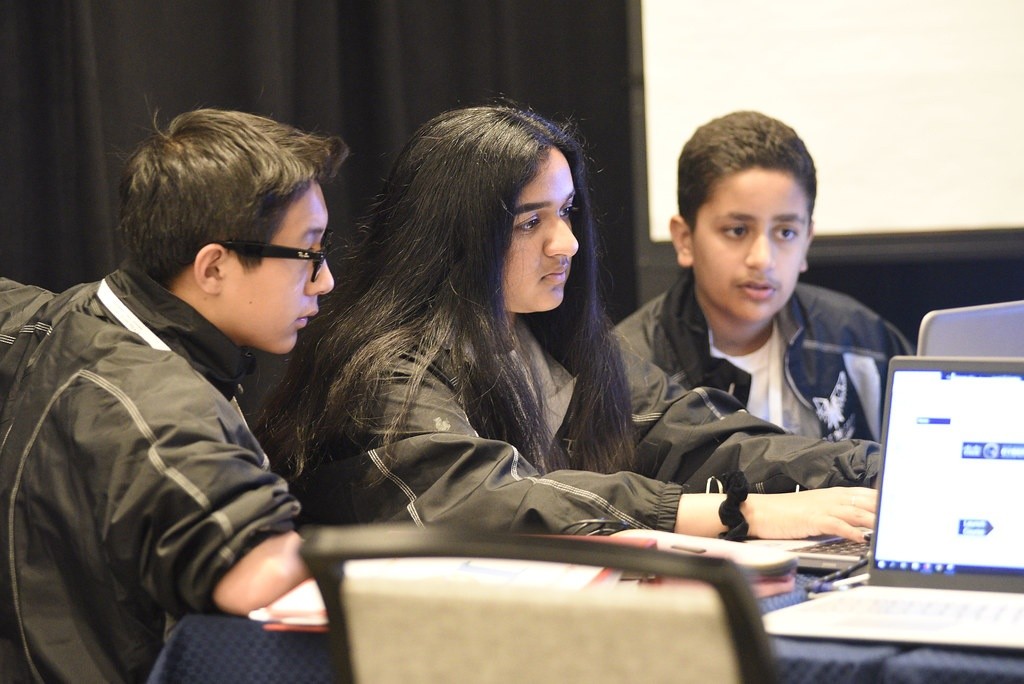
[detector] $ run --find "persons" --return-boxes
[602,110,914,442]
[247,96,879,543]
[0,106,335,683]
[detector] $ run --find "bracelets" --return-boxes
[718,467,751,542]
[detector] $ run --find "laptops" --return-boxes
[734,301,1024,577]
[759,356,1024,654]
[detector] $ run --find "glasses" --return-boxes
[175,231,335,289]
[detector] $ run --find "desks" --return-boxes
[174,537,1023,682]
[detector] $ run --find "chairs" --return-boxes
[299,529,779,684]
